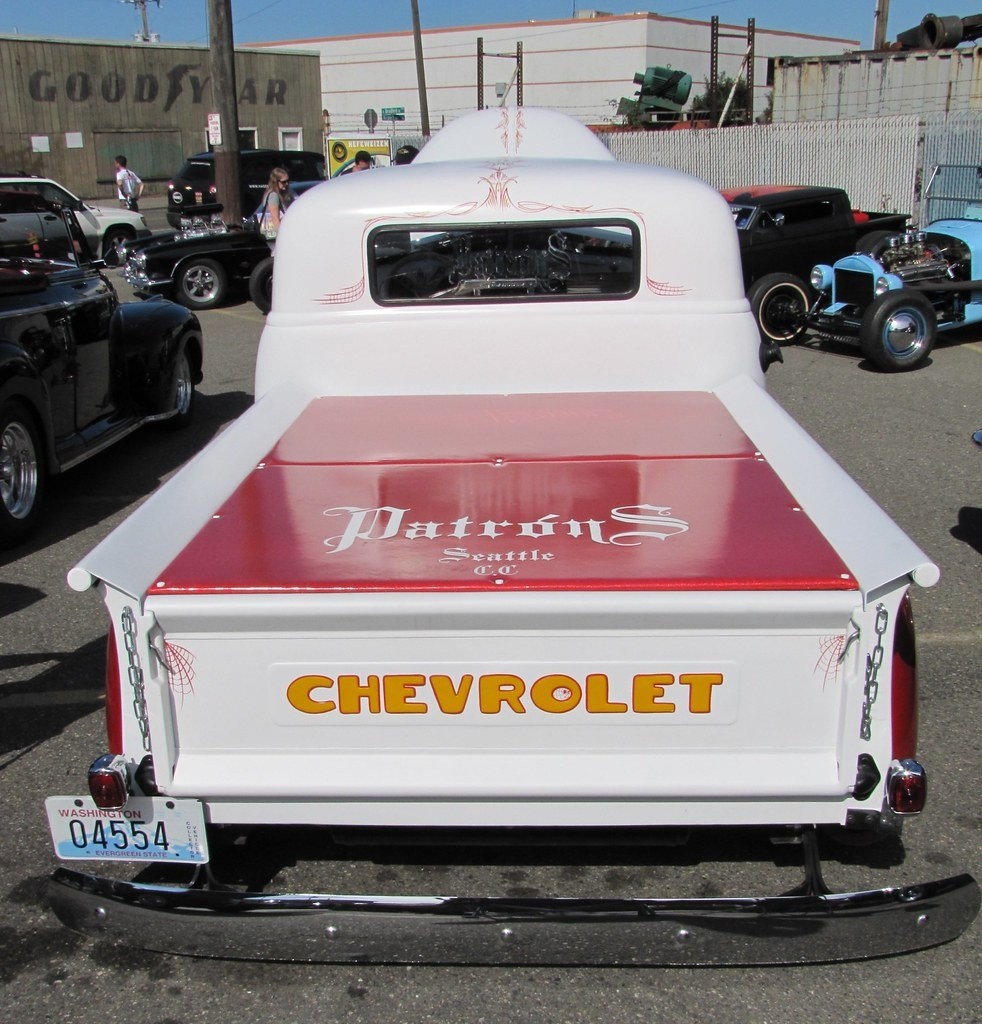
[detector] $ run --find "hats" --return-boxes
[395,145,419,165]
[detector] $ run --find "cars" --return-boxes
[0,178,153,269]
[747,162,982,373]
[1,190,203,541]
[116,181,325,310]
[167,149,326,230]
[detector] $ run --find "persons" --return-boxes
[262,167,289,255]
[114,156,144,213]
[395,145,419,164]
[341,151,371,175]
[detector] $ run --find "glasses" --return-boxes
[279,181,289,183]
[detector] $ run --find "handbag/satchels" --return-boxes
[254,191,287,240]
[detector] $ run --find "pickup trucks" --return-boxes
[719,186,912,302]
[47,108,981,967]
